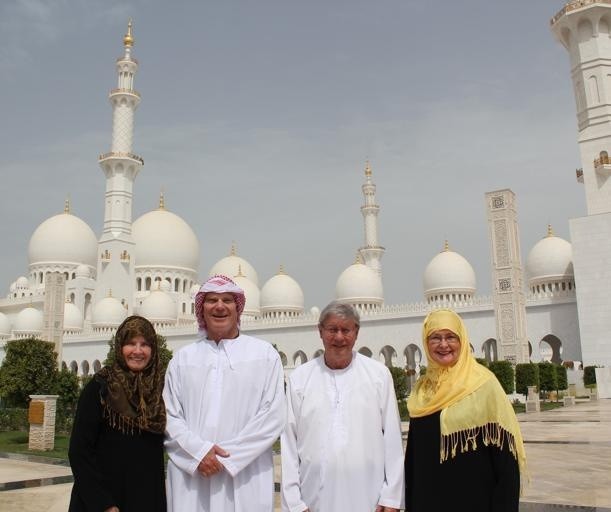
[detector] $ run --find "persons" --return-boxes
[404,309,521,511]
[67,314,172,511]
[281,304,406,510]
[163,272,290,509]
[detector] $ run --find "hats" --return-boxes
[193,275,245,329]
